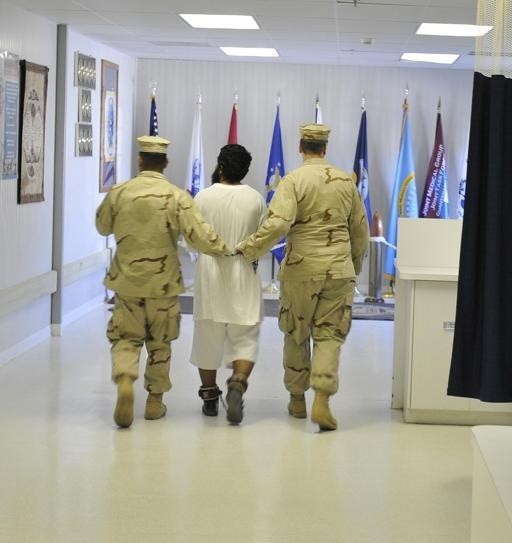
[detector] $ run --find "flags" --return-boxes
[261,105,287,265]
[149,95,159,136]
[350,109,372,228]
[228,103,238,143]
[315,105,323,124]
[420,113,450,219]
[456,149,467,221]
[185,104,204,262]
[382,105,420,281]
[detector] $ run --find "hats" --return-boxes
[299,122,332,140]
[136,136,172,154]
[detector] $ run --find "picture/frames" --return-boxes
[98,58,119,194]
[16,59,50,205]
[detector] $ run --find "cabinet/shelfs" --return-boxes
[389,216,512,427]
[468,423,512,543]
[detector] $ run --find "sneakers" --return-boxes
[225,386,243,423]
[202,397,220,416]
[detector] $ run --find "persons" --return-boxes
[234,124,371,429]
[95,132,234,429]
[191,143,268,426]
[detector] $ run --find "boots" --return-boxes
[310,390,337,430]
[287,393,307,418]
[143,392,167,420]
[113,375,135,427]
